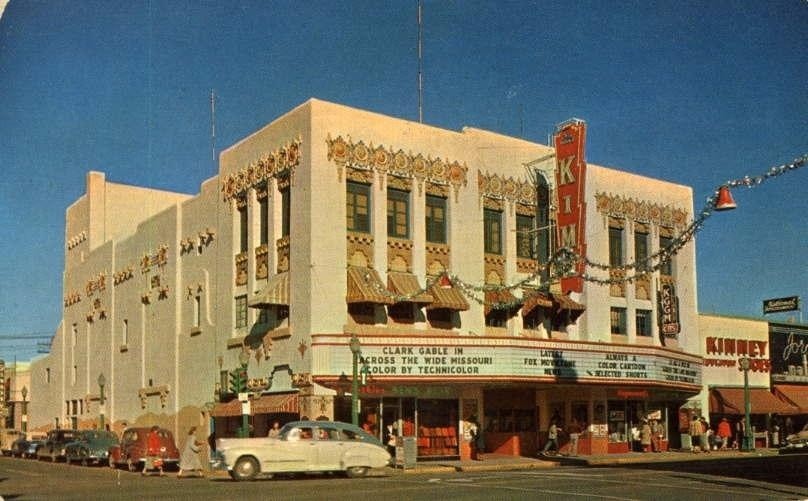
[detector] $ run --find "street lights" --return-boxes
[21,387,27,432]
[742,355,752,437]
[98,372,106,429]
[350,337,360,426]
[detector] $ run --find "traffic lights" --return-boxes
[229,371,248,397]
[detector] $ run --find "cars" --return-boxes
[0,428,79,463]
[107,427,180,472]
[215,419,391,482]
[65,430,119,466]
[778,424,808,455]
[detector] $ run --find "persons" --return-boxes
[541,419,562,456]
[631,417,664,453]
[176,426,209,479]
[118,423,127,442]
[286,415,329,441]
[383,420,398,457]
[267,421,280,437]
[564,417,583,457]
[470,422,485,461]
[679,408,691,434]
[688,416,733,454]
[141,425,167,476]
